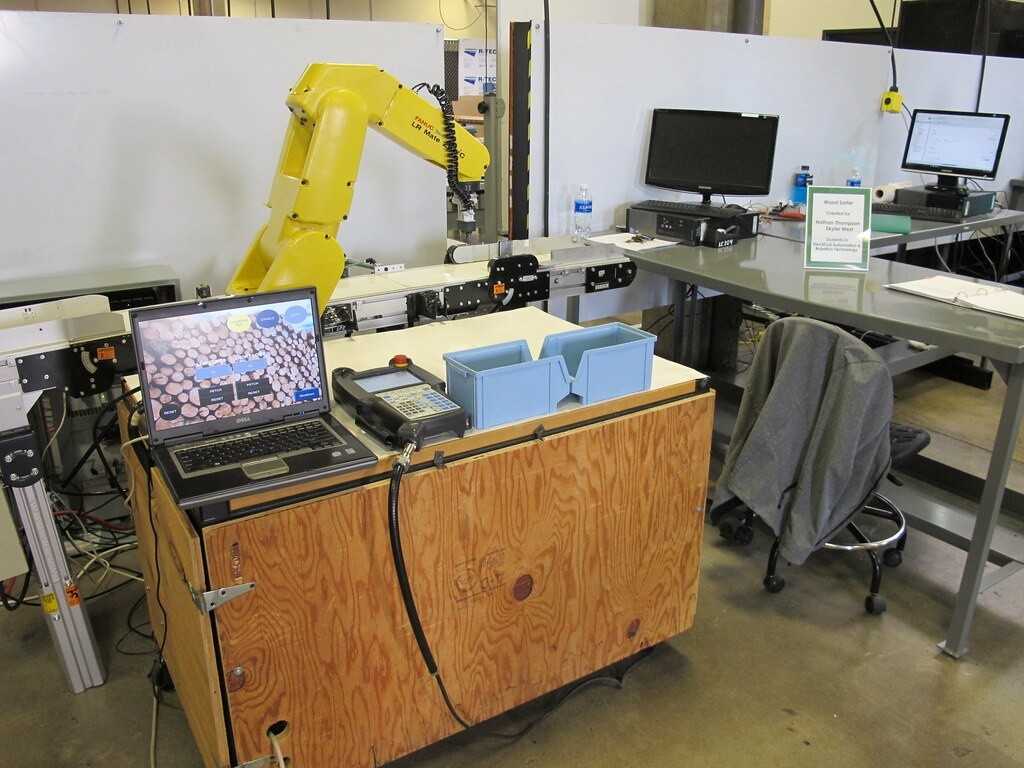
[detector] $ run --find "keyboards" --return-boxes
[630,200,747,219]
[872,202,962,223]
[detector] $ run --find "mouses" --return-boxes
[954,184,969,197]
[724,204,747,213]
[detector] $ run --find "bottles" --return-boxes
[792,166,813,207]
[573,184,592,245]
[845,167,861,187]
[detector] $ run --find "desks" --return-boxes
[2,263,182,556]
[110,305,715,767]
[589,199,1024,658]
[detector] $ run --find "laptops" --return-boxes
[128,285,379,509]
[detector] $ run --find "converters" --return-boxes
[149,660,176,692]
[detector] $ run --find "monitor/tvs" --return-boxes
[901,109,1010,192]
[645,108,779,204]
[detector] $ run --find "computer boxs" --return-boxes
[626,208,758,246]
[893,184,997,218]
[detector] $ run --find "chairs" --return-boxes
[709,317,928,613]
